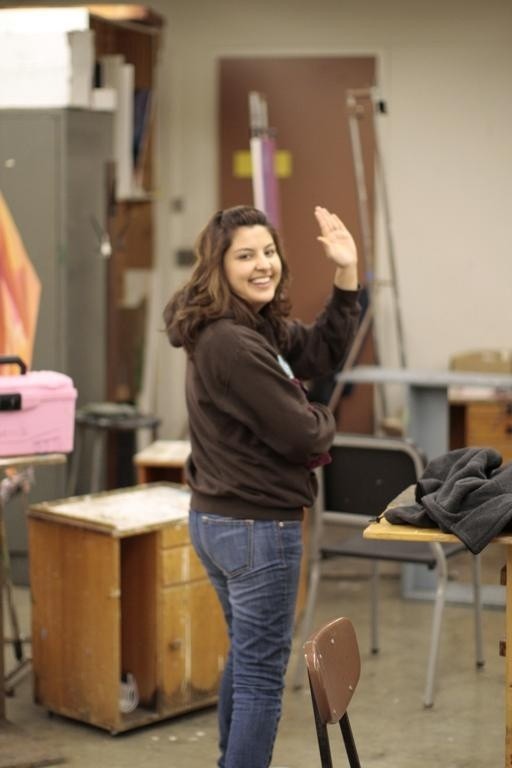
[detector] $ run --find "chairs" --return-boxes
[301,614,363,768]
[290,430,486,711]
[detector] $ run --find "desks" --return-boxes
[0,452,68,721]
[375,472,512,766]
[30,478,230,738]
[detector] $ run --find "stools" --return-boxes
[65,407,162,495]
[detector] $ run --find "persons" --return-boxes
[162,202,369,766]
